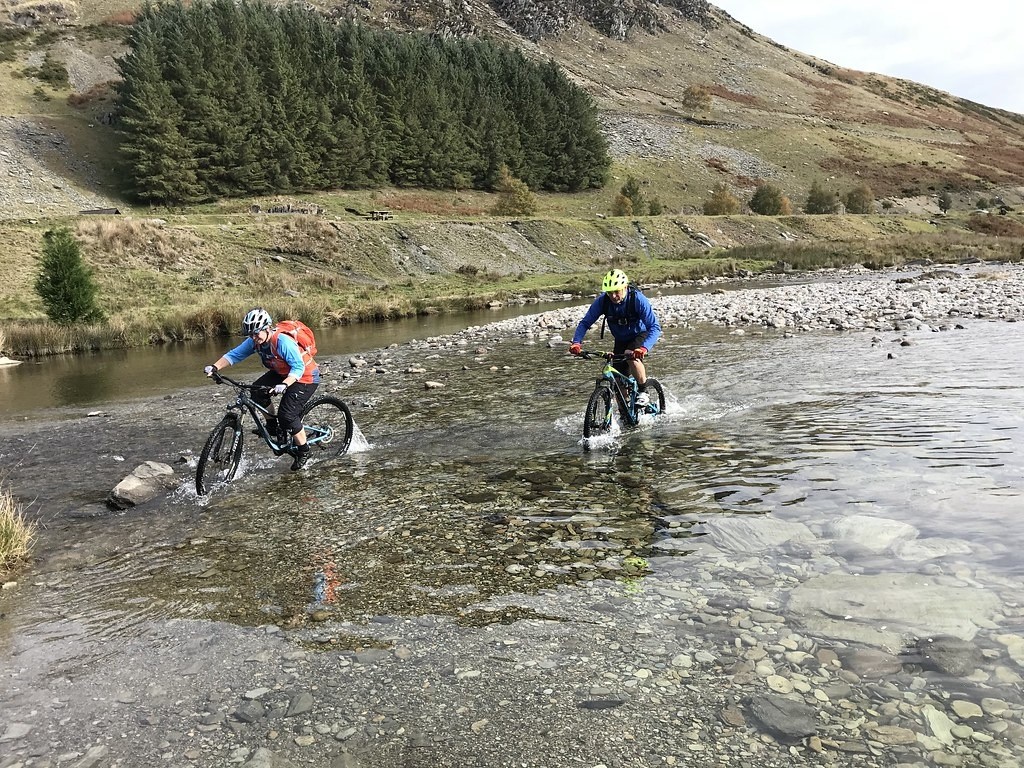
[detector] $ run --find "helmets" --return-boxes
[242,309,272,336]
[601,269,628,293]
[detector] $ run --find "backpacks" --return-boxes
[256,320,318,363]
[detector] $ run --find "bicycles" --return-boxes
[196,369,354,496]
[568,349,666,451]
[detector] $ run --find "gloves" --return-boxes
[630,347,648,360]
[269,384,287,394]
[204,365,218,376]
[570,343,581,356]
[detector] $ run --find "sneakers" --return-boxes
[635,392,650,407]
[290,446,314,471]
[252,426,278,435]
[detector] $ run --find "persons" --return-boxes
[569,269,661,422]
[204,309,320,471]
